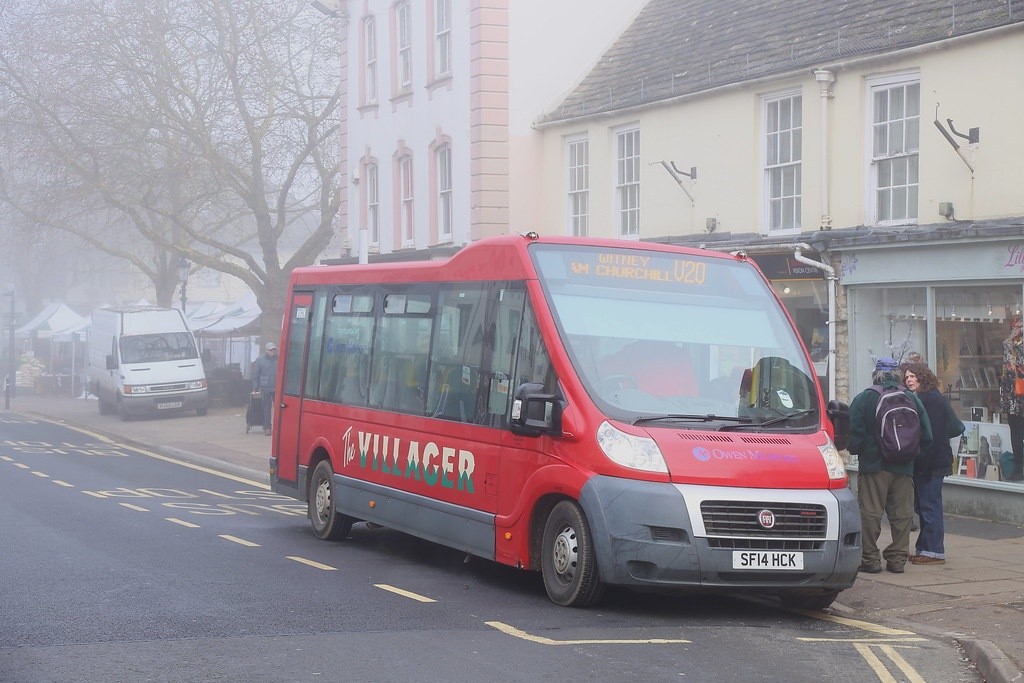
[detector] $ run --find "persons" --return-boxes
[252,342,279,435]
[901,361,955,566]
[999,315,1024,480]
[845,356,935,572]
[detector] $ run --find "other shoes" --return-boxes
[858,564,882,573]
[886,562,905,573]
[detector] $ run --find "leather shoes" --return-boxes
[908,555,920,561]
[265,428,272,436]
[911,555,945,564]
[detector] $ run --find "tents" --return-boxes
[14,299,89,398]
[185,297,261,383]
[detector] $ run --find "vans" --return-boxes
[86,305,208,420]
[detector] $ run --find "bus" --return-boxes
[268,231,864,612]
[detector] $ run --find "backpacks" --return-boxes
[865,384,921,461]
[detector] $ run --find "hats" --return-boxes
[265,343,277,350]
[874,357,899,370]
